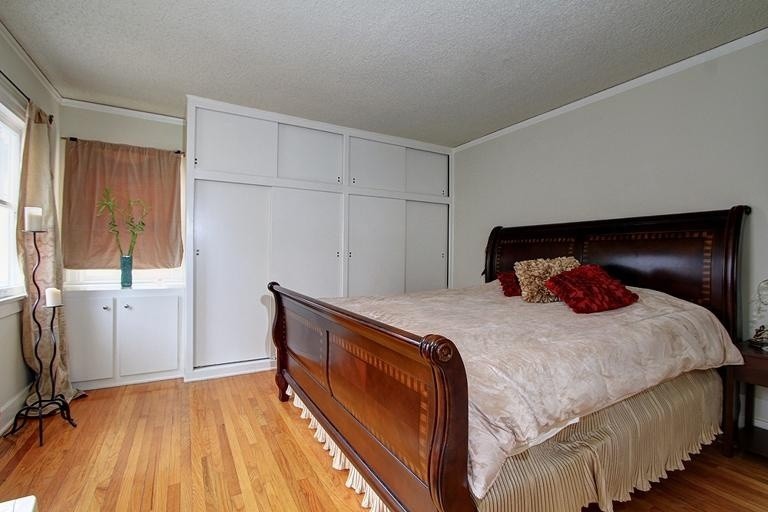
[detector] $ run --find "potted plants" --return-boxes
[95,186,150,286]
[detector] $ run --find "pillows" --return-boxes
[495,255,638,314]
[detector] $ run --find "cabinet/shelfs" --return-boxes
[186,93,346,191]
[347,123,454,203]
[183,177,346,381]
[347,188,449,293]
[59,287,182,392]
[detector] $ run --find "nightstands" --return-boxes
[725,341,768,458]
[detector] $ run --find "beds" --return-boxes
[267,205,752,512]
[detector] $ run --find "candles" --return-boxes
[46,287,61,306]
[24,206,43,232]
